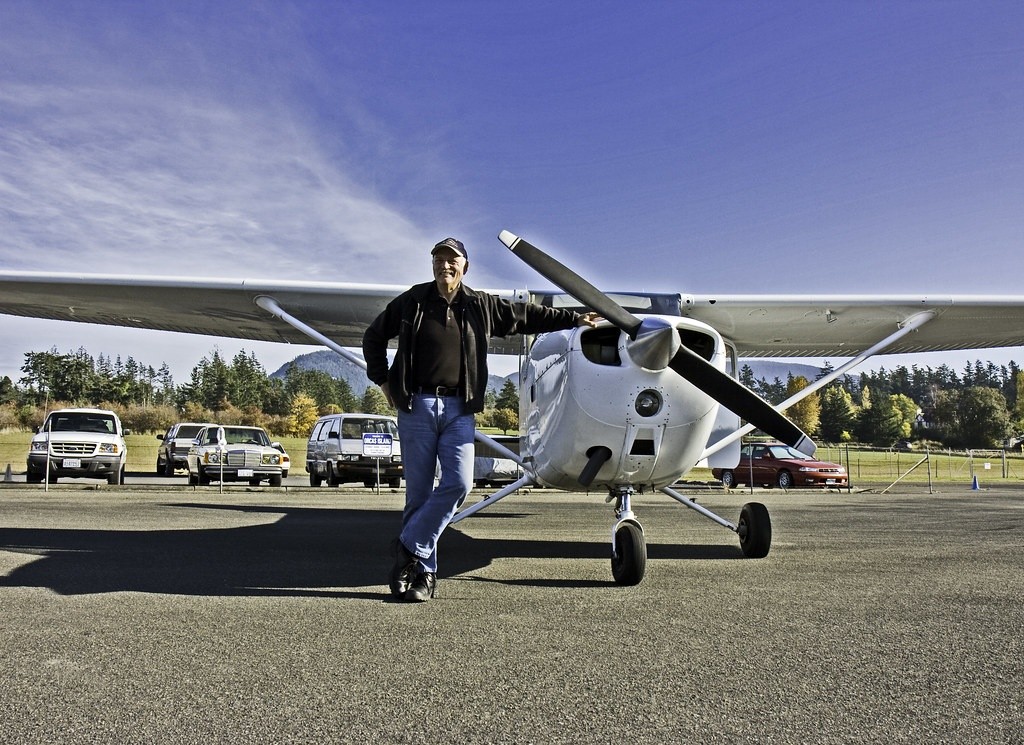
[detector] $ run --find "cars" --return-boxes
[271,441,291,478]
[712,442,848,490]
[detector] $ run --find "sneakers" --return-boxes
[404,571,435,602]
[388,538,419,599]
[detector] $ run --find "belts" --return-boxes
[411,385,465,398]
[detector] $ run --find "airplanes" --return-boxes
[0,231,1024,587]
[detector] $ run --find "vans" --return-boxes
[305,412,404,488]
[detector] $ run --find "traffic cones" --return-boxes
[972,475,980,490]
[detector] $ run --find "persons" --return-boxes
[362,237,600,601]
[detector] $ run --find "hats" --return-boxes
[431,238,467,261]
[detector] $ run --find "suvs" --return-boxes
[157,422,219,478]
[26,407,131,486]
[186,424,284,487]
